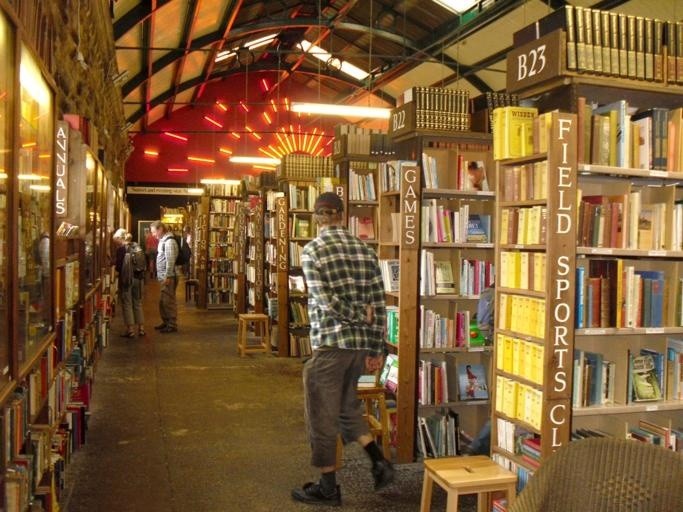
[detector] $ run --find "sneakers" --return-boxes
[154,324,177,333]
[120,330,135,338]
[369,458,396,489]
[291,479,341,505]
[138,327,145,335]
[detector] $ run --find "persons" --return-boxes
[114,228,148,339]
[290,192,397,506]
[150,220,179,334]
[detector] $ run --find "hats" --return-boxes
[114,229,126,238]
[314,192,344,214]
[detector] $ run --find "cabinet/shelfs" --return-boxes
[0,0,129,512]
[178,29,683,512]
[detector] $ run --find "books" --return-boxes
[277,153,332,357]
[192,171,279,348]
[387,86,522,458]
[1,113,114,512]
[333,124,397,448]
[493,5,682,512]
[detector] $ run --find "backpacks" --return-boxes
[162,234,191,265]
[124,241,147,274]
